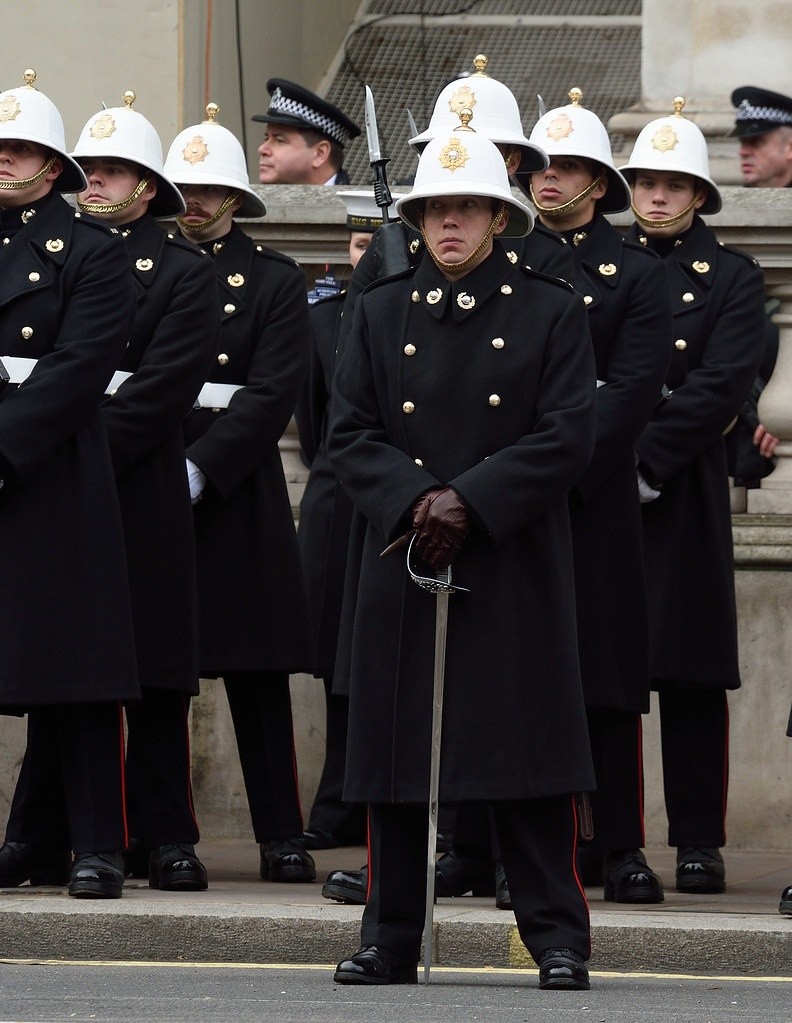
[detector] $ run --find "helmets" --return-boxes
[617,95,722,215]
[0,68,89,191]
[68,90,187,220]
[407,55,549,174]
[163,102,266,217]
[394,108,534,239]
[530,86,632,215]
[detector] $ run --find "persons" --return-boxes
[334,110,602,988]
[723,85,792,188]
[583,99,768,903]
[0,52,792,989]
[108,102,311,885]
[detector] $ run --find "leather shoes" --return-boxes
[597,848,667,902]
[302,827,332,849]
[0,841,69,887]
[321,864,368,903]
[148,844,210,890]
[333,944,419,985]
[539,948,594,989]
[434,862,514,910]
[259,838,316,883]
[71,845,128,900]
[675,847,727,894]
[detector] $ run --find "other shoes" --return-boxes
[779,882,792,918]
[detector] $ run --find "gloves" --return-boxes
[403,485,491,573]
[183,458,207,500]
[630,471,662,505]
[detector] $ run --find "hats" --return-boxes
[726,84,792,138]
[249,78,361,148]
[335,190,410,233]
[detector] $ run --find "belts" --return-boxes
[0,355,39,384]
[195,383,247,409]
[105,369,137,396]
